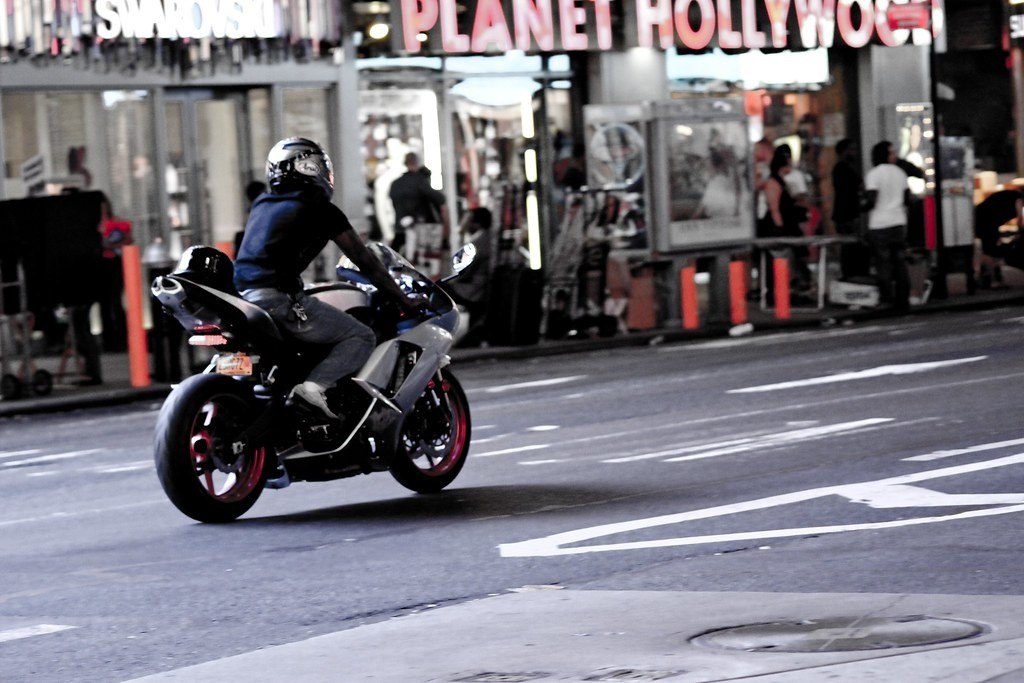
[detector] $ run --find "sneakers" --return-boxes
[288,384,338,423]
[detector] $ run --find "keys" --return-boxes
[292,304,308,330]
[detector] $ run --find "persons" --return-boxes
[750,112,932,312]
[227,138,427,425]
[391,152,495,348]
[32,179,266,389]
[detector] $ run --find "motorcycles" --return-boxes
[150,241,478,525]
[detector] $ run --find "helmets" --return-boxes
[265,137,334,200]
